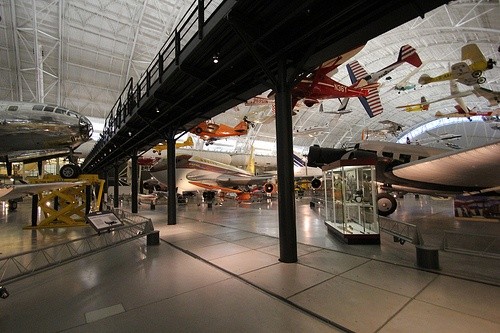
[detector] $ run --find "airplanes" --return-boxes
[191,115,254,147]
[0,100,94,180]
[361,118,405,143]
[305,137,499,216]
[318,97,353,122]
[268,55,379,112]
[144,154,274,208]
[153,136,194,155]
[337,43,422,120]
[380,43,500,122]
